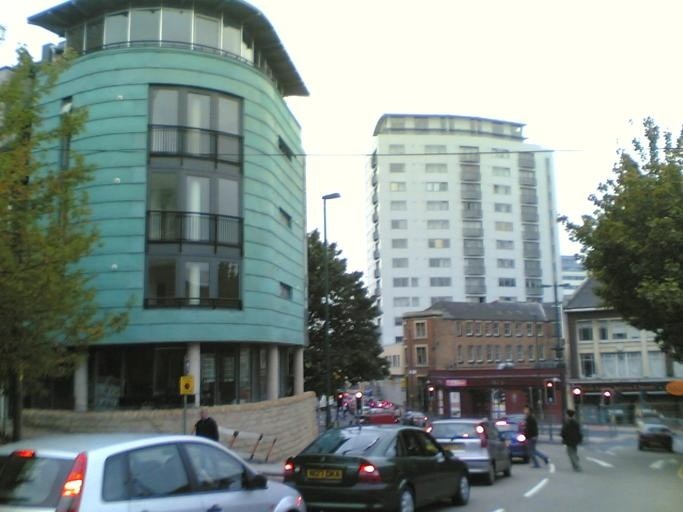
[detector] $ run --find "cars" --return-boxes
[636,423,678,452]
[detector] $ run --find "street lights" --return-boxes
[322,192,340,426]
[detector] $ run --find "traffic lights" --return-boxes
[427,384,436,401]
[337,393,344,407]
[571,386,582,410]
[544,379,557,405]
[355,392,363,415]
[599,388,613,409]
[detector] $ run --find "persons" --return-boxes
[195,409,219,442]
[520,404,549,468]
[559,410,584,472]
